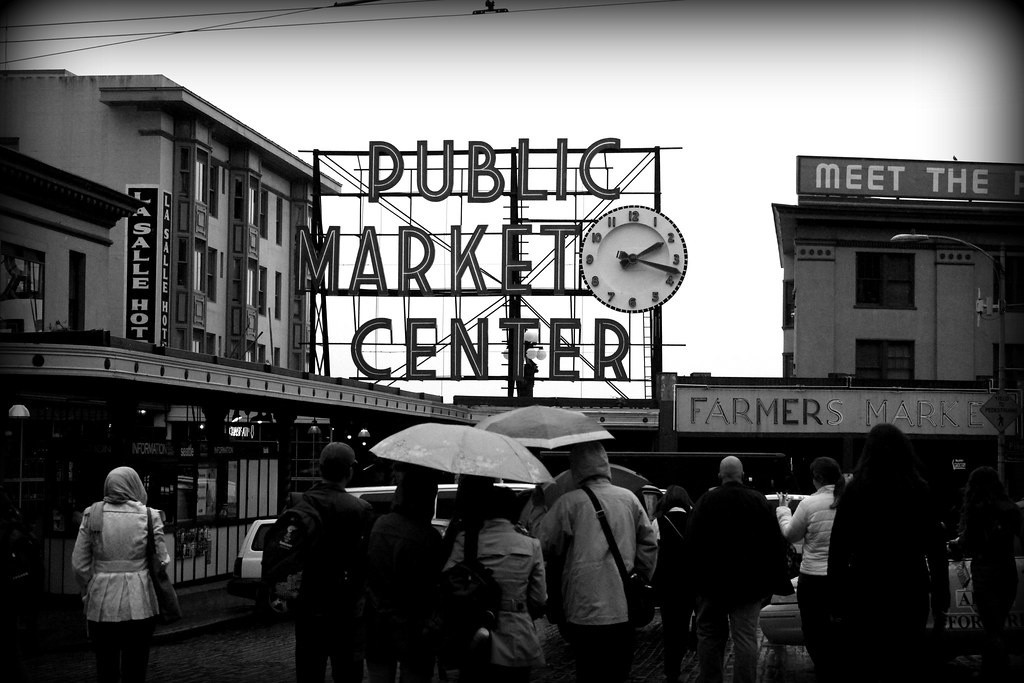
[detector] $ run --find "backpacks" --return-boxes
[440,522,503,637]
[264,491,326,580]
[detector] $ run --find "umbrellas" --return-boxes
[519,464,650,530]
[370,422,555,484]
[474,404,615,486]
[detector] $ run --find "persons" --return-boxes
[649,483,694,683]
[947,466,1024,673]
[442,472,549,683]
[527,440,659,683]
[292,441,371,683]
[827,423,951,683]
[71,466,170,683]
[364,470,444,683]
[776,456,846,683]
[685,455,783,683]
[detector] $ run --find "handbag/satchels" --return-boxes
[146,506,182,627]
[625,569,656,629]
[783,537,801,581]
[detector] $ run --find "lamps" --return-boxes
[308,416,322,434]
[249,408,270,424]
[7,401,32,417]
[358,421,371,438]
[232,410,242,423]
[263,410,277,424]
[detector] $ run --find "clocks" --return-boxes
[578,206,688,313]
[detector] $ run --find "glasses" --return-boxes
[348,460,358,467]
